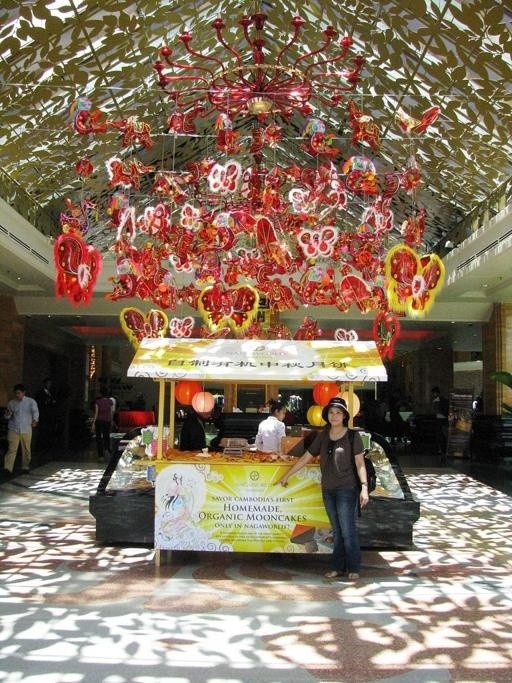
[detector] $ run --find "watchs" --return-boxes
[360,482,368,485]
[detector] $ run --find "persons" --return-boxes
[89,389,114,461]
[106,392,117,423]
[176,406,215,450]
[253,398,287,452]
[272,396,377,583]
[35,376,54,403]
[1,383,41,475]
[431,386,445,452]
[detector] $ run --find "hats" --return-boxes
[322,397,350,424]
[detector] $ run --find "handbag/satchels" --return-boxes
[348,430,377,495]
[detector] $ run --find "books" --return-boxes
[446,391,476,446]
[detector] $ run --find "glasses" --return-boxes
[328,441,336,454]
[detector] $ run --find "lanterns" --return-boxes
[191,390,215,413]
[175,380,200,404]
[339,391,361,415]
[307,404,327,426]
[314,382,341,406]
